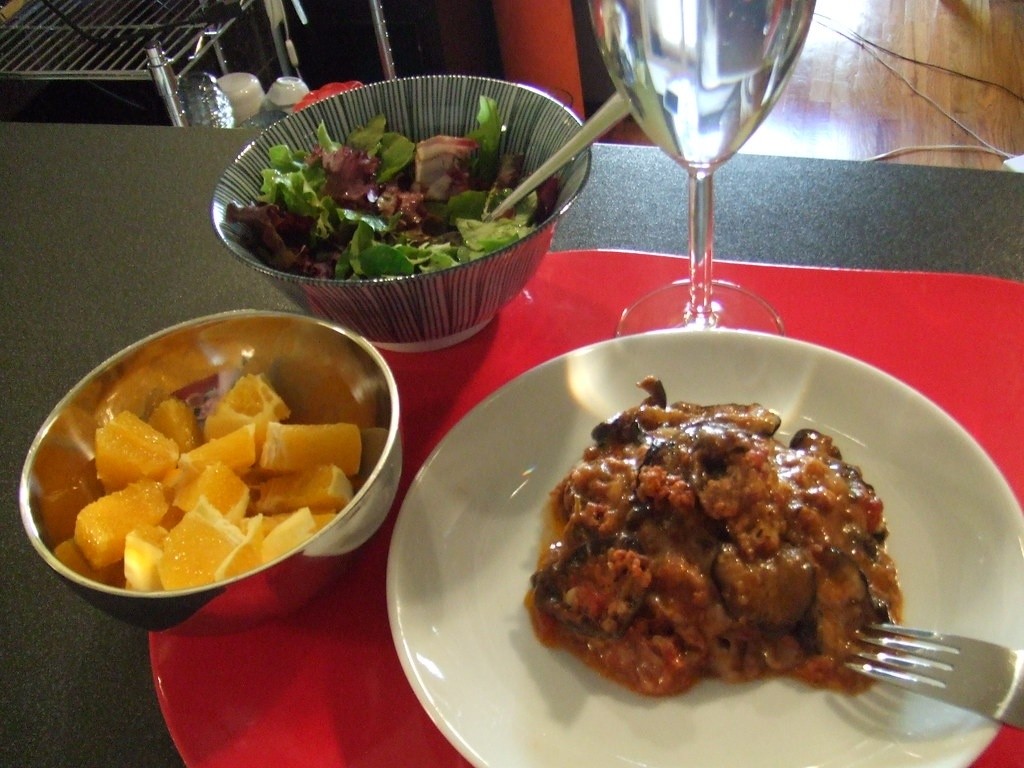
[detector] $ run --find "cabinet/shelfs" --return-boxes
[1,1,399,129]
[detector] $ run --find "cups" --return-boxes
[265,77,310,115]
[217,72,265,127]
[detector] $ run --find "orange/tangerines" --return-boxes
[71,370,364,592]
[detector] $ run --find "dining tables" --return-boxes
[0,122,1024,768]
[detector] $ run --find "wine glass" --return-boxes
[587,0,818,338]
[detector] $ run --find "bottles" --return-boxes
[174,72,235,129]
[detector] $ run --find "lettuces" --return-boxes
[223,91,563,281]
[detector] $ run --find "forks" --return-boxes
[842,623,1024,732]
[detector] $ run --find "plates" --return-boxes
[385,327,1024,768]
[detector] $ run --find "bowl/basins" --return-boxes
[17,308,399,636]
[208,76,592,352]
